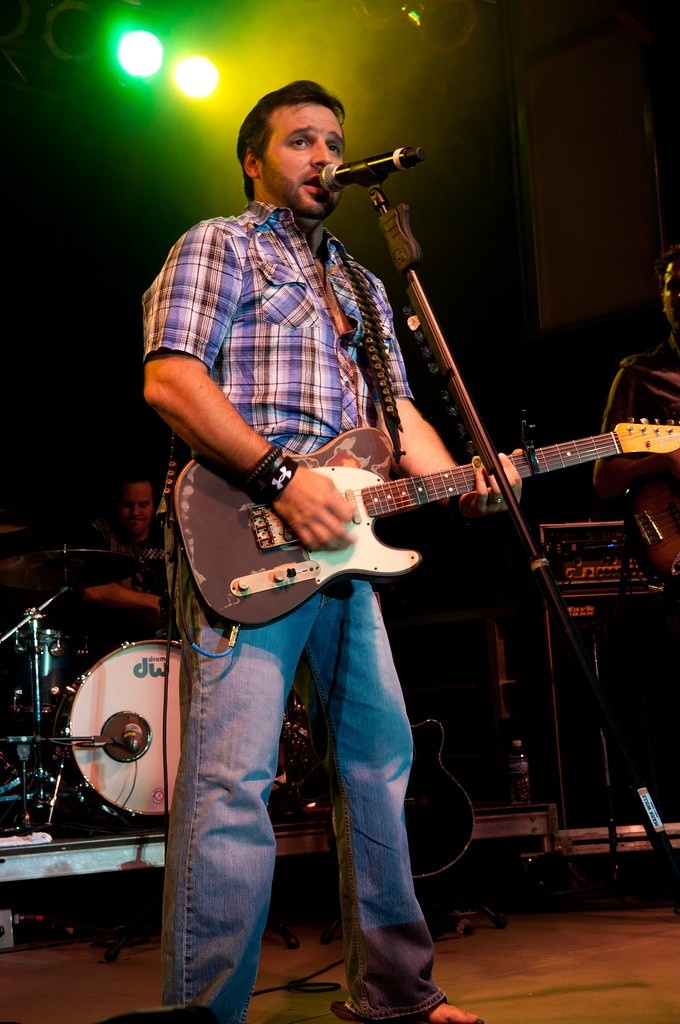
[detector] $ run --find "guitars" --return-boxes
[172,417,680,628]
[626,469,680,576]
[402,719,475,880]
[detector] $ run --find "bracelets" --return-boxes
[241,445,299,508]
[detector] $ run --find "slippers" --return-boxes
[331,1001,484,1024]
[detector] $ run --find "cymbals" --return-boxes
[0,549,143,591]
[0,524,27,533]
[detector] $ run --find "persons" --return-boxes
[84,476,162,641]
[592,244,680,914]
[142,80,524,1024]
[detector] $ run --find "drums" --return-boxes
[54,639,186,819]
[12,629,88,715]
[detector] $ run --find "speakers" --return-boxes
[526,586,680,830]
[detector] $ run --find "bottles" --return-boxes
[507,740,531,804]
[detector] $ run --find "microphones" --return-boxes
[319,146,426,194]
[123,723,143,754]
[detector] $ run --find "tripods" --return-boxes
[0,556,130,832]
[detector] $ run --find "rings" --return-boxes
[492,497,504,504]
